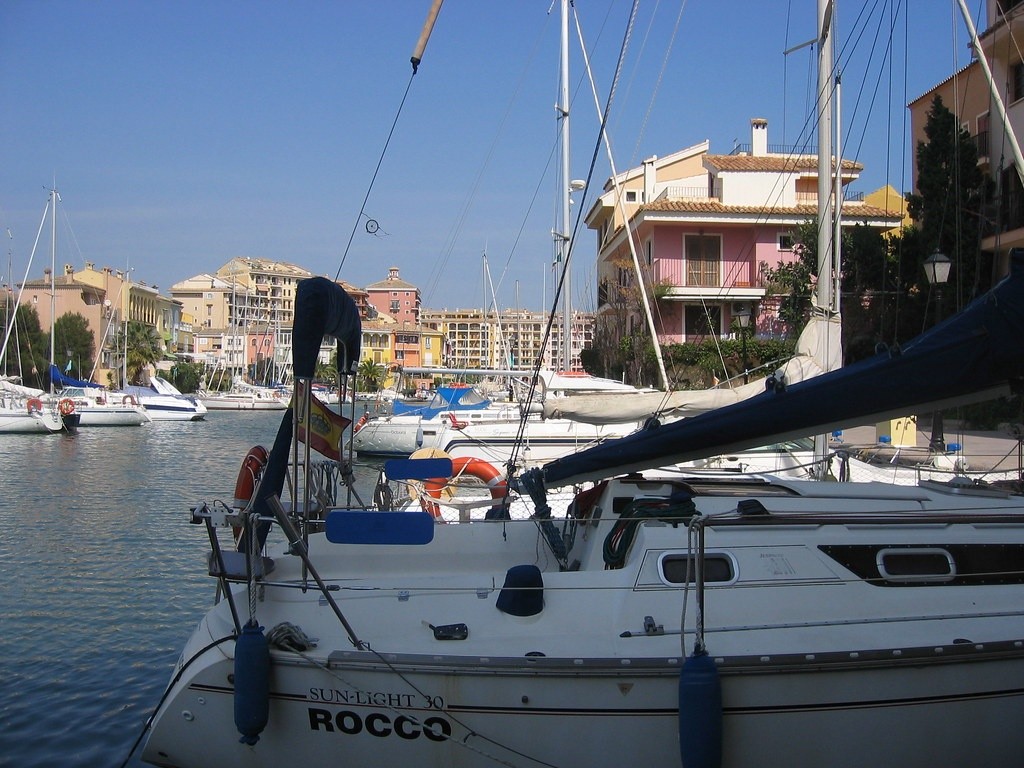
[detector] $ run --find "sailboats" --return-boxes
[0,233,65,434]
[1,173,151,427]
[122,2,1024,768]
[51,269,207,421]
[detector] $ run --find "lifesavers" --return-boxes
[59,398,75,414]
[230,443,269,554]
[418,454,514,526]
[26,398,43,414]
[352,414,368,434]
